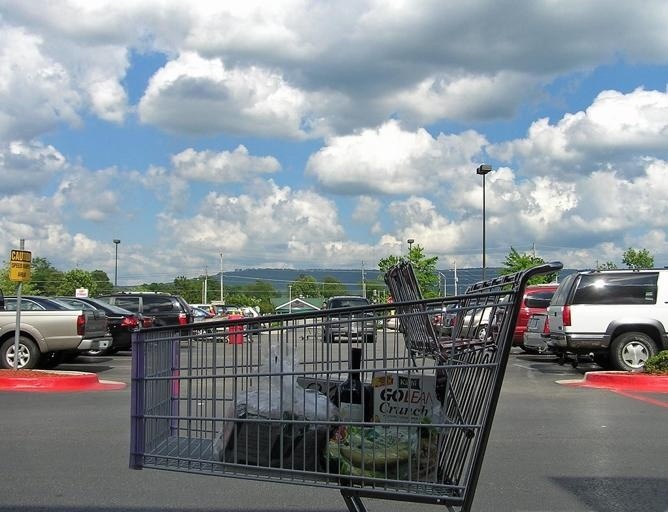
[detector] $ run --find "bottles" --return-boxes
[338,347,369,426]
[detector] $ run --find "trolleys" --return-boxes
[125,259,564,510]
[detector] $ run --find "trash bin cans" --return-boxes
[228,314,245,344]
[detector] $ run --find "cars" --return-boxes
[0,291,262,370]
[386,267,667,373]
[321,295,377,343]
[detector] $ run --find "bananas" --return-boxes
[324,427,412,484]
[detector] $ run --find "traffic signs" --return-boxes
[9,249,31,282]
